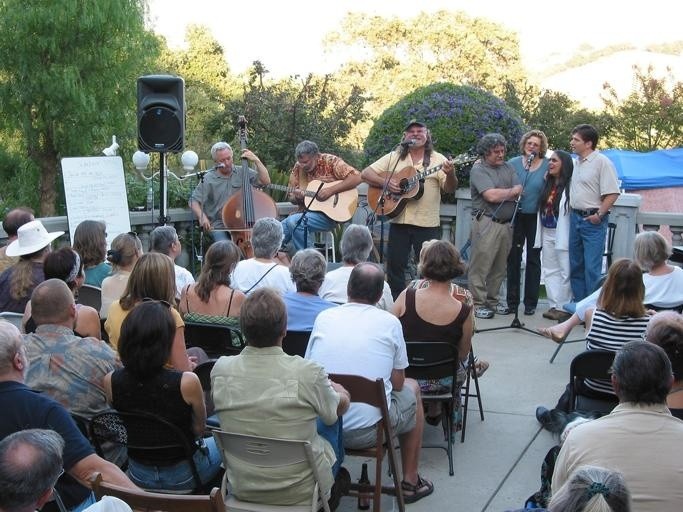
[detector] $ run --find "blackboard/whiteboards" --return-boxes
[60,156,132,264]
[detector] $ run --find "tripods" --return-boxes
[474,159,544,336]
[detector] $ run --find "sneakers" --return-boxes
[489,303,508,315]
[473,305,494,319]
[537,406,549,427]
[543,308,572,323]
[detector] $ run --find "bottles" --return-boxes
[147,187,154,212]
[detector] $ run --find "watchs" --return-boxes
[596,211,604,219]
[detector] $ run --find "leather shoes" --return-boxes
[525,307,535,315]
[508,304,517,313]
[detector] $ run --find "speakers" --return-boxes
[137,75,184,154]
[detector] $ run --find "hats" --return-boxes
[5,219,65,257]
[403,118,425,132]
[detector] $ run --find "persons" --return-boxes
[188,143,270,242]
[533,150,574,321]
[506,129,550,316]
[361,118,459,303]
[281,140,364,250]
[567,124,620,325]
[641,310,682,418]
[539,339,682,511]
[537,231,683,342]
[0,207,489,512]
[537,259,657,432]
[467,134,523,319]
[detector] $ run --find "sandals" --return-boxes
[402,474,434,505]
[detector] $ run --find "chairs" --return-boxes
[645,303,682,315]
[569,350,619,415]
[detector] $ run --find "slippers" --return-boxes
[470,360,489,379]
[535,327,563,344]
[425,413,443,426]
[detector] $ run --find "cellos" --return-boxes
[222,116,277,259]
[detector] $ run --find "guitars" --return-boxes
[262,181,359,223]
[366,154,477,218]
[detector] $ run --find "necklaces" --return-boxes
[667,388,682,395]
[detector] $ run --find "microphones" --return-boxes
[215,163,226,169]
[527,151,537,162]
[401,139,416,145]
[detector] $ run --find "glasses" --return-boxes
[142,297,171,310]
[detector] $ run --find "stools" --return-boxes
[310,228,335,264]
